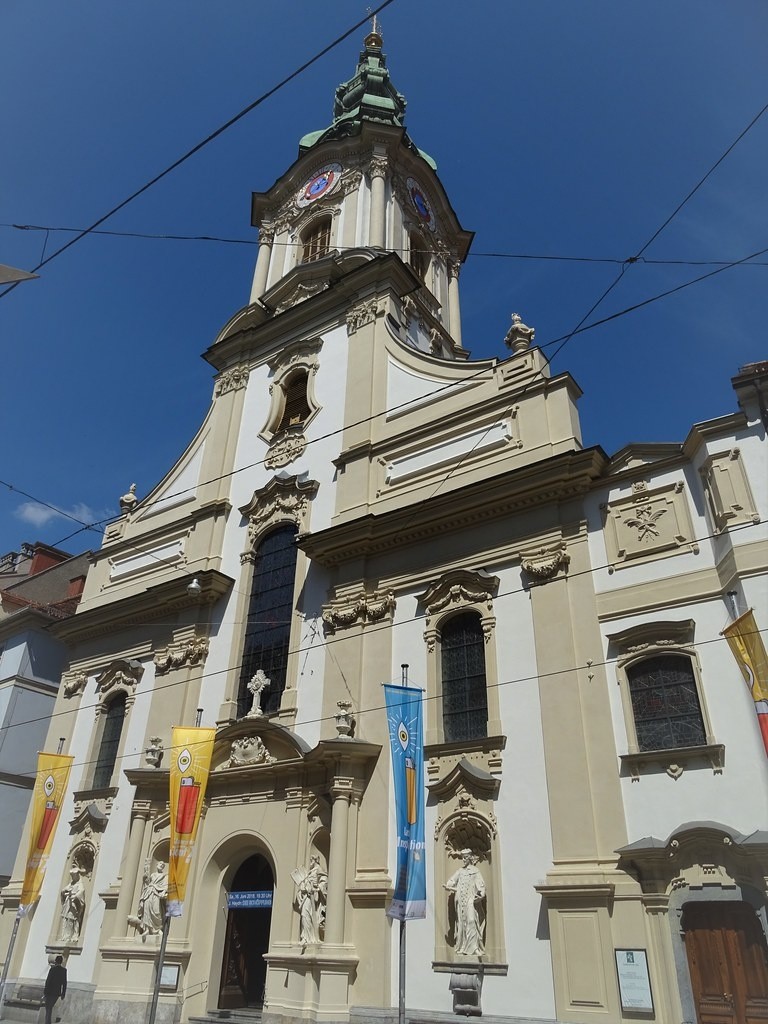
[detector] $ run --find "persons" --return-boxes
[44,956,67,1024]
[138,861,168,937]
[61,868,85,941]
[445,849,487,956]
[297,855,328,943]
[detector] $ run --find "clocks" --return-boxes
[297,162,343,209]
[406,176,436,232]
